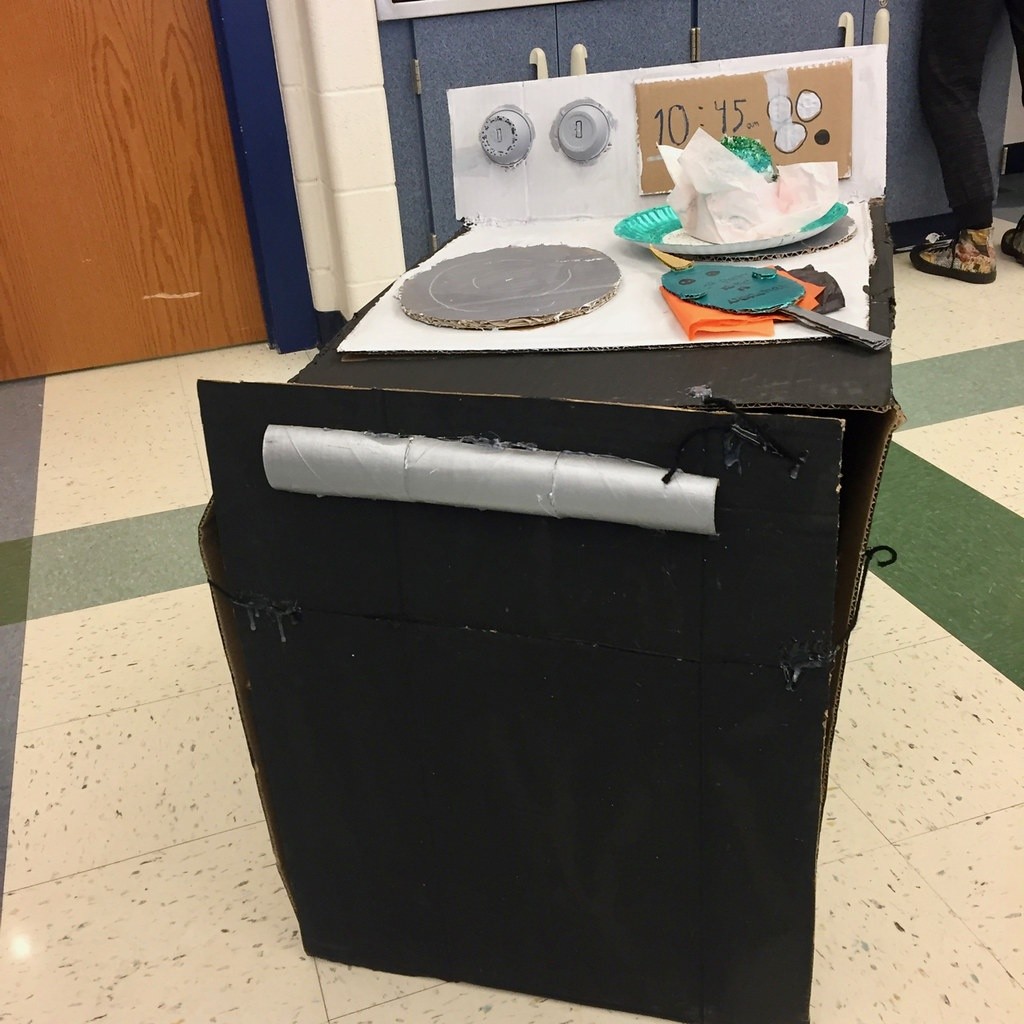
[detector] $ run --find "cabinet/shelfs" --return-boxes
[379,0,696,271]
[696,0,1015,249]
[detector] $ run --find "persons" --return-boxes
[909,0,1024,284]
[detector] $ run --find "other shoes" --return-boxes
[1001,214,1024,268]
[910,227,998,283]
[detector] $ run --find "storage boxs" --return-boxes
[196,197,905,1024]
